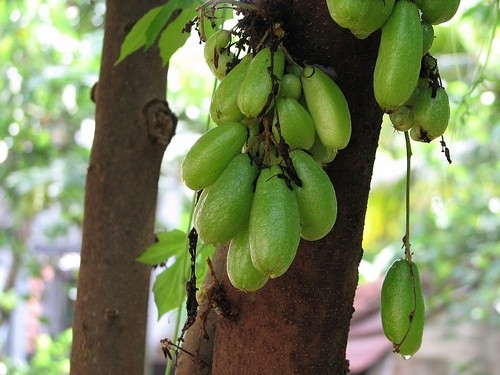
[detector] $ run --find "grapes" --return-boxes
[325,0,461,142]
[182,29,352,293]
[381,258,424,354]
